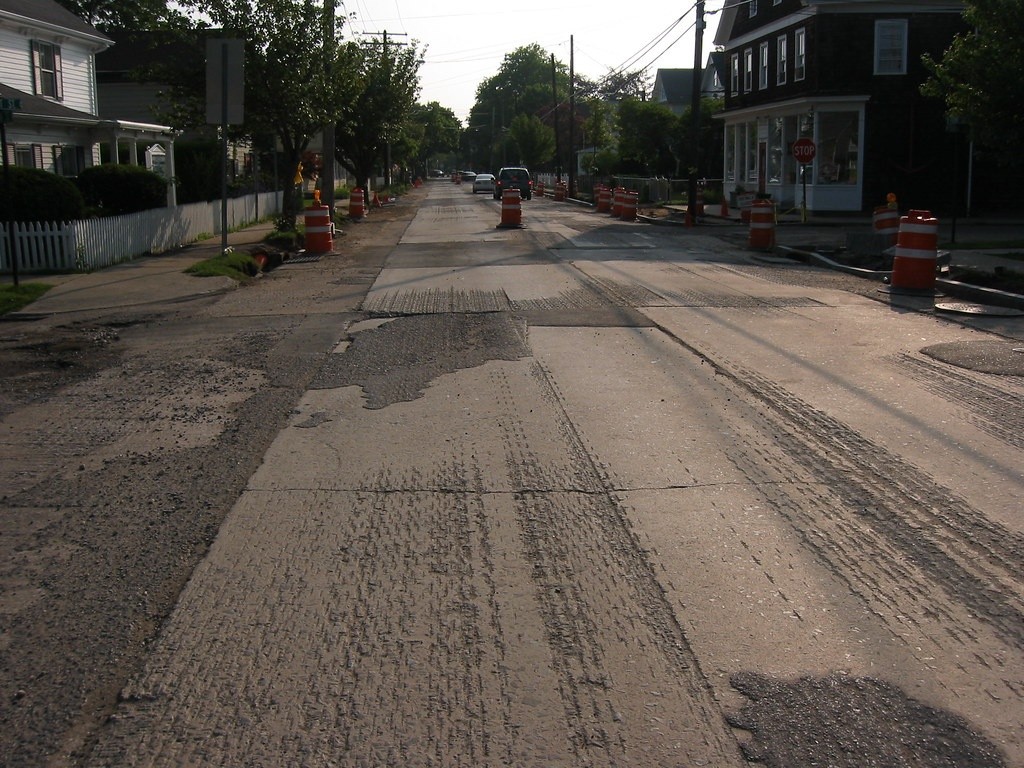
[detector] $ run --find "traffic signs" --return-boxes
[0,96,22,110]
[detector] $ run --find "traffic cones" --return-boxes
[373,194,381,207]
[720,197,729,216]
[684,209,693,227]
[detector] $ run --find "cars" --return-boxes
[472,173,496,194]
[461,171,477,182]
[491,167,532,200]
[434,170,444,177]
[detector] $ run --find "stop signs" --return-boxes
[793,138,816,163]
